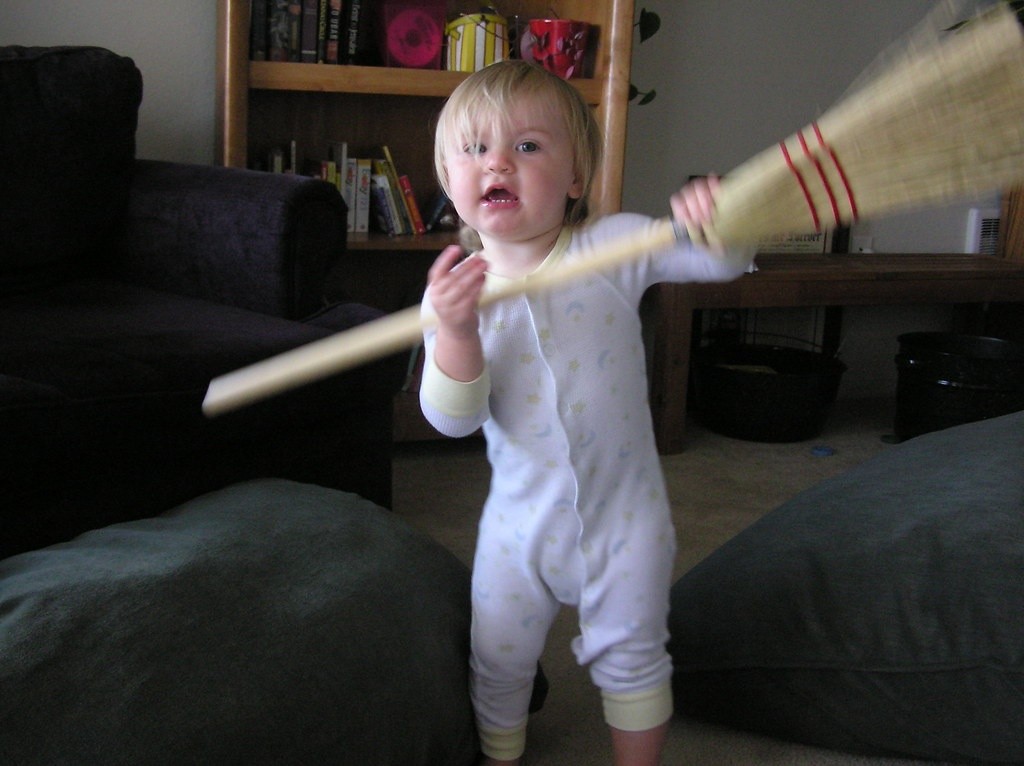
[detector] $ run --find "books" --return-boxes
[262,140,447,235]
[252,0,368,66]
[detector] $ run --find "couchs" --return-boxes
[0,45,396,559]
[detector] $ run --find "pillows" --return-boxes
[677,409,1024,766]
[0,481,550,766]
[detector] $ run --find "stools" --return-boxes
[881,331,1024,442]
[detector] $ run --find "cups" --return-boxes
[528,18,590,80]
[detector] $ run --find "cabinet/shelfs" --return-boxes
[216,0,635,245]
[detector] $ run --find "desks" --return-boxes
[643,253,1024,432]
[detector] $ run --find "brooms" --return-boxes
[201,0,1024,418]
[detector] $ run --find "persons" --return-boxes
[417,58,760,766]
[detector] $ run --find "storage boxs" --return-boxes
[525,19,600,78]
